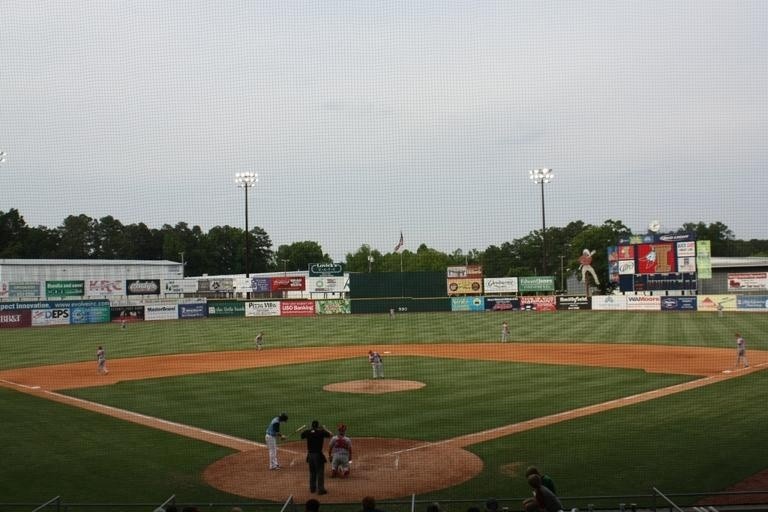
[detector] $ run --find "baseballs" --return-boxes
[349,461,353,464]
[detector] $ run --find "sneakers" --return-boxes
[270,465,350,495]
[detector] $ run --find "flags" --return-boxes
[394,232,402,250]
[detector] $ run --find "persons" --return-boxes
[426,502,442,512]
[305,499,319,512]
[263,411,289,472]
[467,499,499,512]
[501,320,510,342]
[368,350,384,378]
[121,321,126,328]
[578,248,599,286]
[734,333,748,368]
[255,330,266,351]
[359,496,379,512]
[96,346,109,375]
[165,506,243,512]
[300,421,333,495]
[327,423,353,477]
[522,467,580,512]
[389,308,395,321]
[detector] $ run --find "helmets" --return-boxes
[279,413,288,423]
[312,421,319,428]
[337,423,347,432]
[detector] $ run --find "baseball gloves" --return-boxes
[328,455,333,463]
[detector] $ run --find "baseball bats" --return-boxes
[285,424,306,438]
